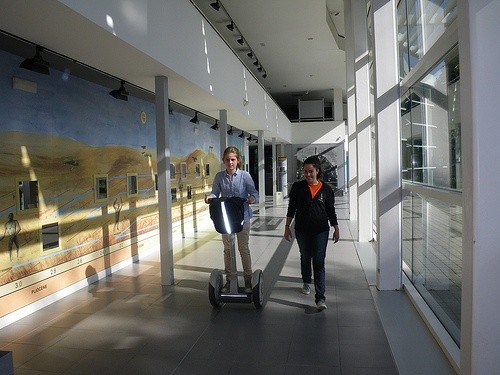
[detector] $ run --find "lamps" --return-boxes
[107,80,129,101]
[253,59,258,66]
[237,36,244,45]
[247,134,252,141]
[167,99,174,114]
[190,111,199,124]
[210,119,218,130]
[19,45,51,75]
[262,71,267,78]
[238,131,245,138]
[210,0,220,11]
[256,139,258,143]
[257,65,262,72]
[226,21,234,31]
[247,50,253,59]
[227,126,233,135]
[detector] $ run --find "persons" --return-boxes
[205,147,261,294]
[285,153,341,313]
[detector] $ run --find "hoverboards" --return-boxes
[206,198,267,309]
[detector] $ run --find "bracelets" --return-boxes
[286,224,289,226]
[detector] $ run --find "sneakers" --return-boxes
[221,280,252,294]
[302,283,312,294]
[317,299,327,311]
